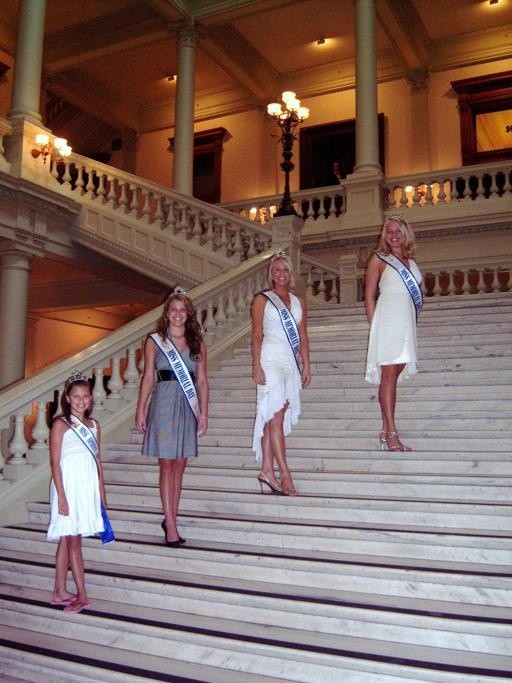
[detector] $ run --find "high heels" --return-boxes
[258,470,284,496]
[379,430,412,451]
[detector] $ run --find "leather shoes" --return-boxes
[162,518,185,547]
[281,481,297,495]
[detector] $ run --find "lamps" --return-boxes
[266,89,322,214]
[165,74,174,82]
[487,0,501,5]
[30,131,72,163]
[406,183,435,196]
[315,39,327,45]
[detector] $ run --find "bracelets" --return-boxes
[201,413,208,419]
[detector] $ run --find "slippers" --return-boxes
[51,593,91,615]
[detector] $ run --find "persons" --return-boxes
[365,208,423,452]
[251,251,311,496]
[44,370,108,612]
[136,288,210,545]
